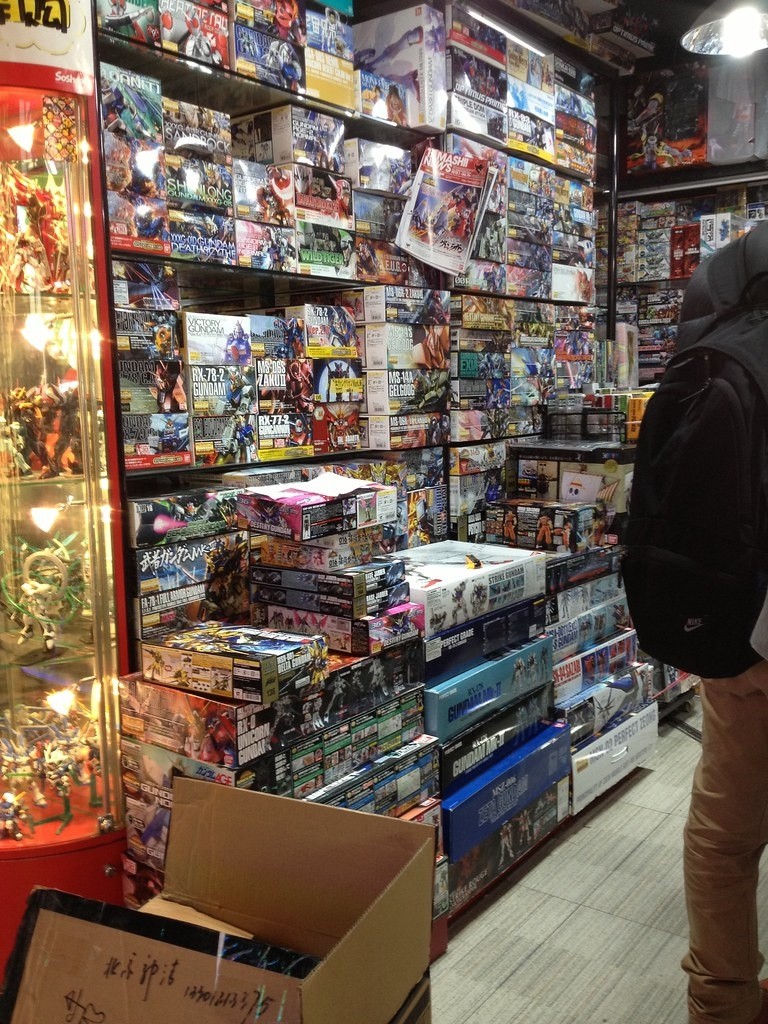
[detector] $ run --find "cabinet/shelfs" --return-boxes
[90,0,768,969]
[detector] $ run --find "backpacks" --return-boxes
[619,234,768,678]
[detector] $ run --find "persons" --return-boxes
[614,214,765,1020]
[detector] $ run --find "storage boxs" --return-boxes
[93,1,767,964]
[0,777,435,1024]
